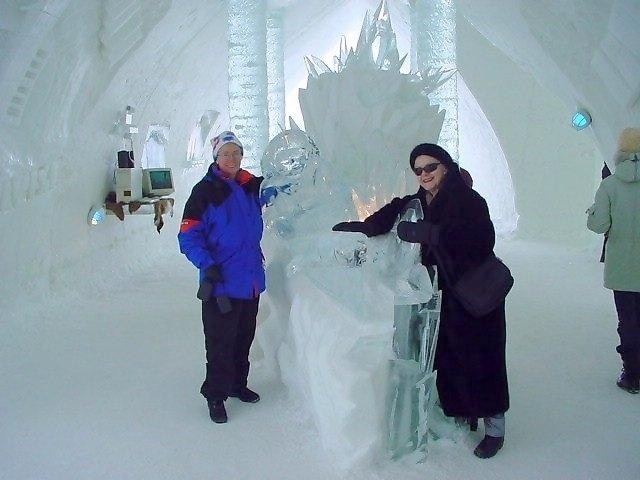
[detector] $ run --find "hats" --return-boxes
[210,131,243,161]
[410,143,453,171]
[619,127,640,153]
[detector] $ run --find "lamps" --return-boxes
[88,203,105,227]
[572,111,592,131]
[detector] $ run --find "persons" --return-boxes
[330,142,515,462]
[175,130,296,423]
[583,126,639,395]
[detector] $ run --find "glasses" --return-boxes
[413,162,441,175]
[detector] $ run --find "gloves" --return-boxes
[332,222,364,232]
[397,219,440,248]
[206,265,223,283]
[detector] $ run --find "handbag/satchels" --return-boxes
[452,253,514,320]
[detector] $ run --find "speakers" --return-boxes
[118,150,134,168]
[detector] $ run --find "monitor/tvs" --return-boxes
[142,167,175,197]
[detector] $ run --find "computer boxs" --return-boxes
[116,168,142,204]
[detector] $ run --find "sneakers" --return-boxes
[474,434,504,458]
[617,372,639,394]
[207,398,228,423]
[227,386,260,403]
